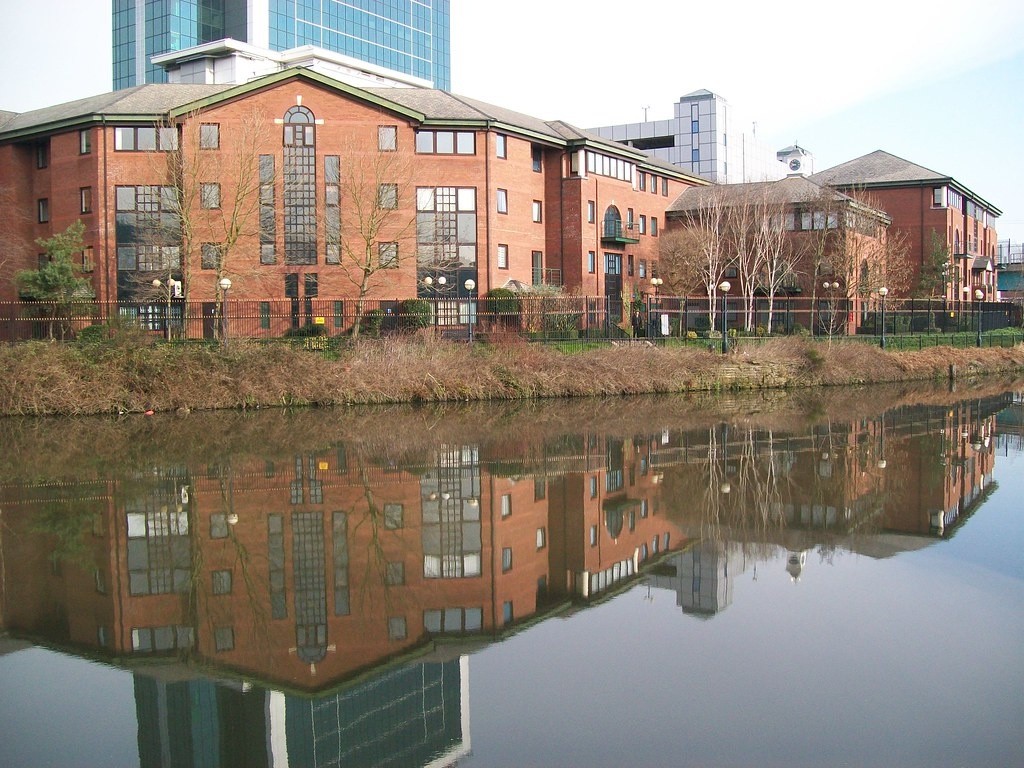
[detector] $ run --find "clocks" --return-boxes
[790,159,800,171]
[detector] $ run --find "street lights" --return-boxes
[963,287,969,332]
[718,281,731,355]
[823,282,839,336]
[975,292,984,349]
[217,278,234,340]
[879,287,888,349]
[649,277,663,338]
[151,278,175,344]
[424,277,447,338]
[464,278,475,344]
